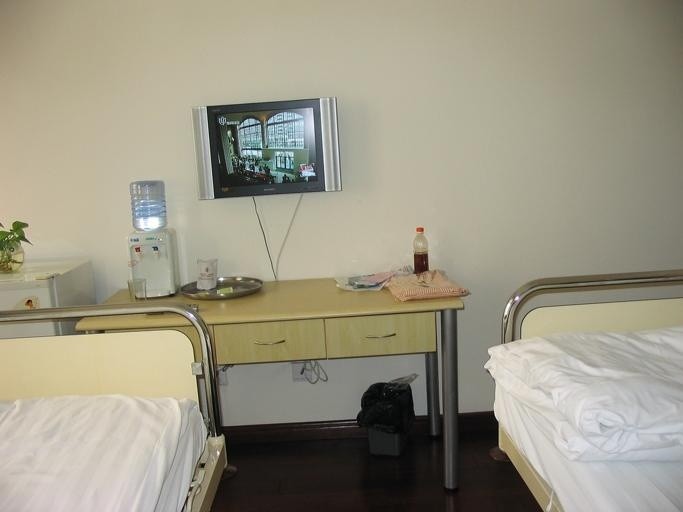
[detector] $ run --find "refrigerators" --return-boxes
[0,259,99,338]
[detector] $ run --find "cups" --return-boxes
[127,279,147,303]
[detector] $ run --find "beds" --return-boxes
[498,269,682,511]
[0,304,230,512]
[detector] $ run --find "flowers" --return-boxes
[76,278,464,490]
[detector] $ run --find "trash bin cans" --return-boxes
[362,382,413,456]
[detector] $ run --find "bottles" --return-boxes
[412,227,429,275]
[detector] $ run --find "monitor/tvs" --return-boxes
[191,96,343,201]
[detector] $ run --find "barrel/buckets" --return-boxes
[128,181,166,231]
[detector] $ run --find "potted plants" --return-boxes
[0,221,33,272]
